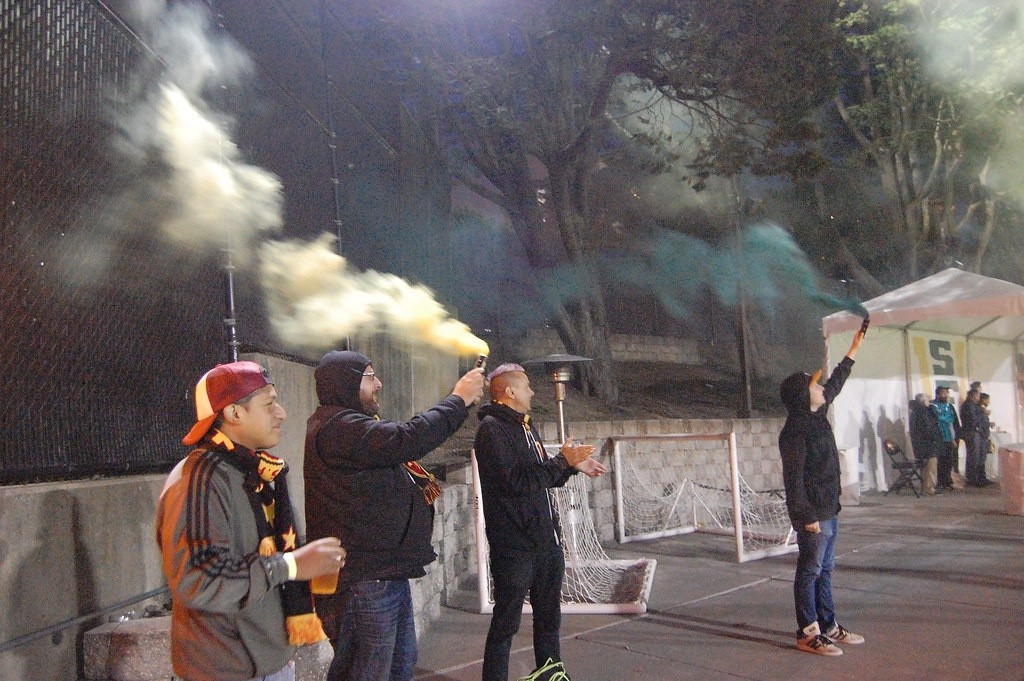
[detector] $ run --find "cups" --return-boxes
[311,553,341,595]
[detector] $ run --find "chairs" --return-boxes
[882,439,929,499]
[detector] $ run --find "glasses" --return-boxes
[362,372,375,381]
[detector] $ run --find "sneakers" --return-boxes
[796,621,844,656]
[820,623,865,645]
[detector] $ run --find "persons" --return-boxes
[470,362,606,679]
[155,362,347,679]
[909,392,941,497]
[929,381,997,488]
[305,351,485,679]
[778,331,867,655]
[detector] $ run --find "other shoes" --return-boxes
[925,480,996,496]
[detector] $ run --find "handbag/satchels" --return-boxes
[987,436,996,454]
[517,655,571,681]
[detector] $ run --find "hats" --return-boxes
[181,359,275,445]
[936,386,949,392]
[804,368,822,388]
